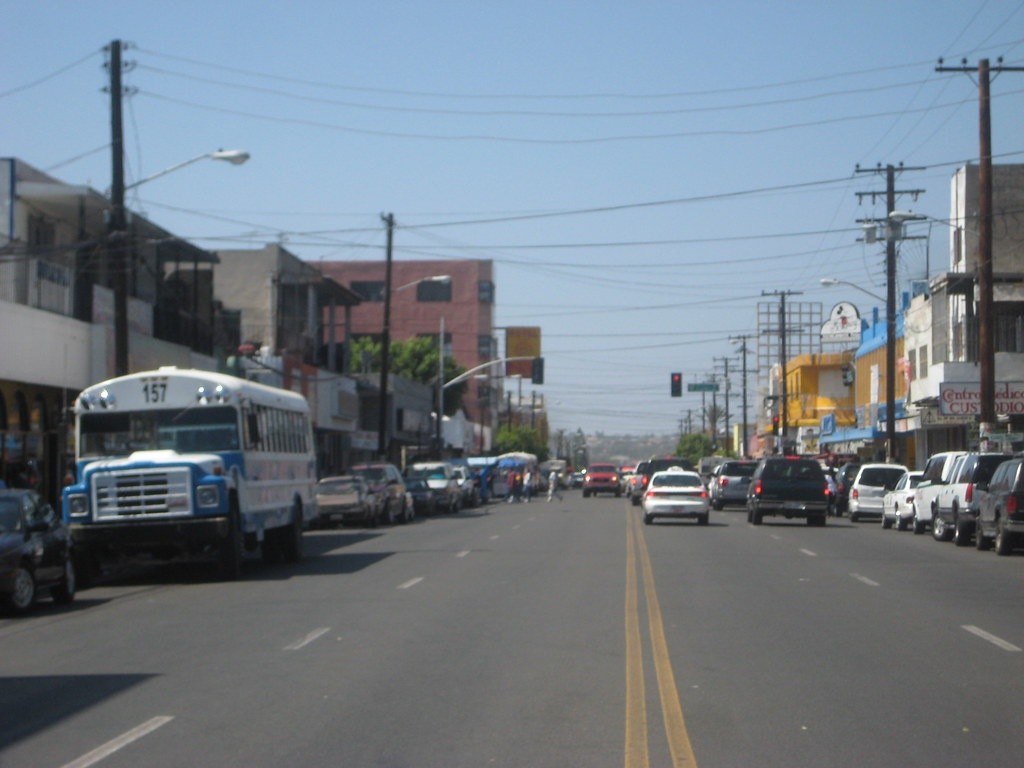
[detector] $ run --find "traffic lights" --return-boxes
[772,416,779,435]
[671,372,682,397]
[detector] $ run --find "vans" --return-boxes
[742,456,830,526]
[622,457,760,511]
[834,462,867,517]
[849,463,910,522]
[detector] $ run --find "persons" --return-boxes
[479,465,492,506]
[547,466,562,503]
[501,467,532,503]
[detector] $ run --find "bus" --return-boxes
[62,364,320,580]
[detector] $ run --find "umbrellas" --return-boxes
[498,457,529,469]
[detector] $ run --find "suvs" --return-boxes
[912,450,1024,556]
[581,462,621,499]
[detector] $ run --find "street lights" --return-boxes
[109,145,251,377]
[378,273,455,461]
[819,277,896,463]
[889,210,995,450]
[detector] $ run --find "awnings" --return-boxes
[819,426,907,445]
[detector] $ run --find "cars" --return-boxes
[0,488,78,613]
[882,470,925,529]
[318,461,475,527]
[641,470,710,525]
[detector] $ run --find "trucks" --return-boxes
[480,451,539,497]
